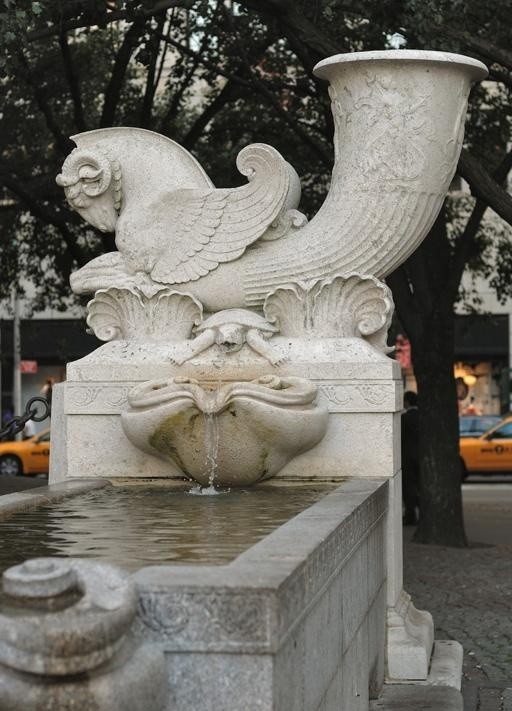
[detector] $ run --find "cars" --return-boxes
[1,429,51,478]
[459,416,512,483]
[459,414,511,438]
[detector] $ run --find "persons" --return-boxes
[400,390,423,524]
[21,417,36,440]
